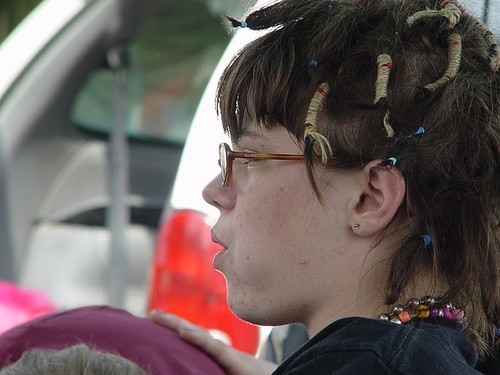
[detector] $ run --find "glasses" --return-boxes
[218,142,344,187]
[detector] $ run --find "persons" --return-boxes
[150,0,500,375]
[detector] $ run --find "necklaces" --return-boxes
[378,295,467,326]
